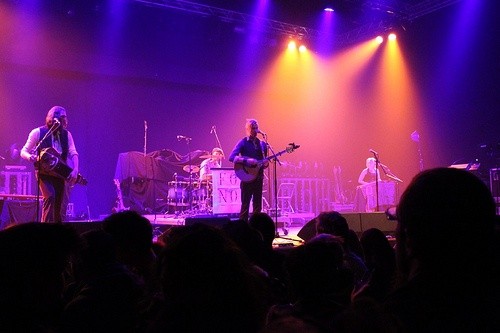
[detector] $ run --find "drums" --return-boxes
[167,181,190,206]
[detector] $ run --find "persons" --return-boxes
[199,148,225,182]
[0,167,499,333]
[358,157,382,185]
[20,105,79,222]
[229,119,269,222]
[0,142,25,165]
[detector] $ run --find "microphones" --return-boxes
[368,148,378,155]
[255,130,266,135]
[177,135,192,140]
[210,125,216,133]
[53,117,61,125]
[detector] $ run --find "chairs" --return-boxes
[65,182,90,220]
[277,183,295,214]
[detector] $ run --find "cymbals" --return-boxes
[183,165,199,172]
[200,155,220,158]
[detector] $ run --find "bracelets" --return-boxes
[242,158,248,163]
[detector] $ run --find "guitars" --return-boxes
[34,147,88,185]
[234,143,300,182]
